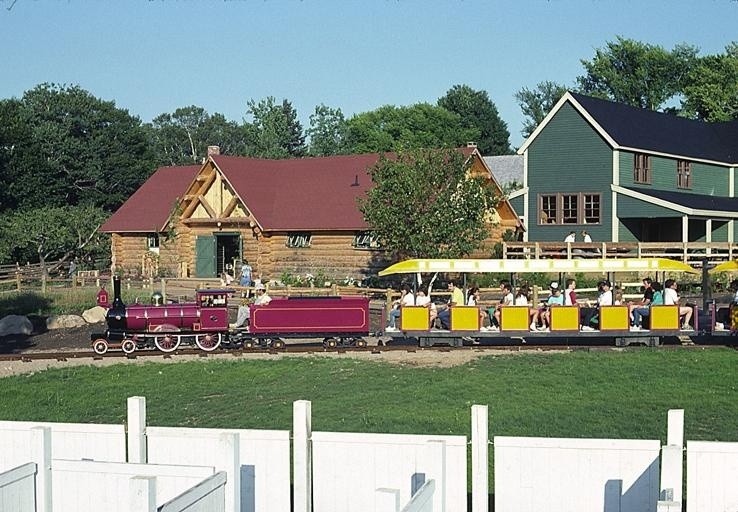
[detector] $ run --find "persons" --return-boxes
[713,278,738,331]
[227,283,272,328]
[220,273,229,287]
[515,282,563,332]
[563,230,576,242]
[468,279,513,331]
[579,230,593,242]
[385,279,464,331]
[564,278,612,332]
[616,277,693,330]
[237,260,253,298]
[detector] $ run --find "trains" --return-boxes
[86,254,738,359]
